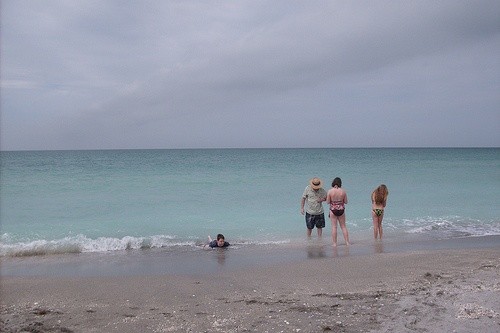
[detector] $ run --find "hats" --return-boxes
[309,177,323,189]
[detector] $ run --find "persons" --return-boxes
[300,177,328,240]
[208,234,231,248]
[327,176,350,247]
[371,185,389,241]
